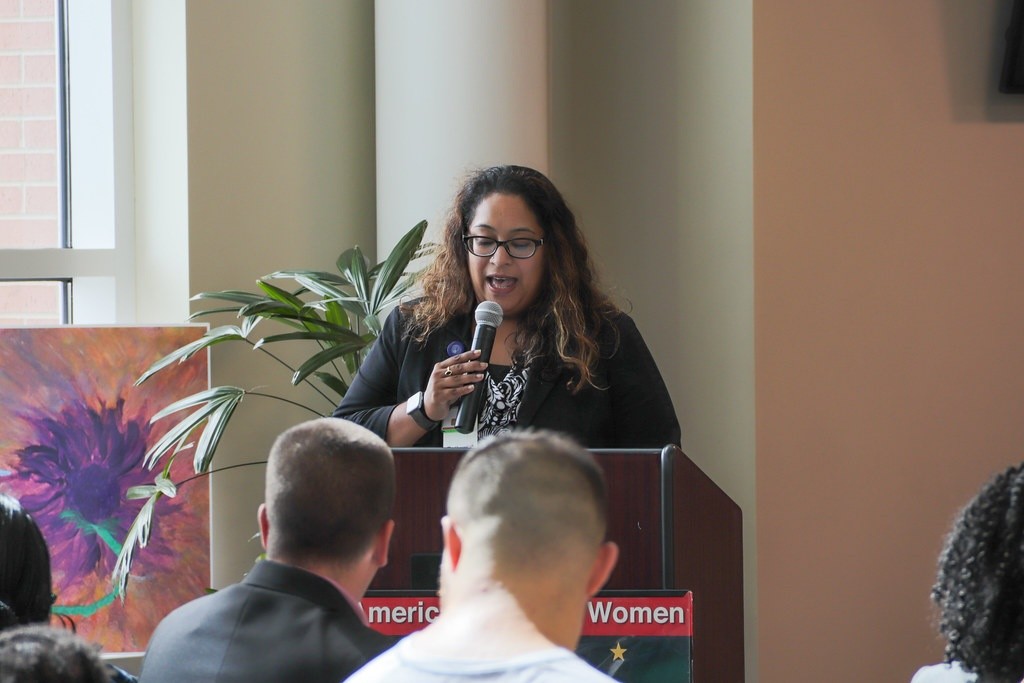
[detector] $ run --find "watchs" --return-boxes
[407,390,440,432]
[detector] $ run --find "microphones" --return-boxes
[455,301,504,434]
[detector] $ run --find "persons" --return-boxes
[342,431,620,682]
[138,418,408,682]
[1,494,140,683]
[909,461,1024,682]
[332,164,681,448]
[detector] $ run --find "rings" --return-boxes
[445,365,452,376]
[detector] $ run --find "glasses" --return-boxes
[461,228,545,259]
